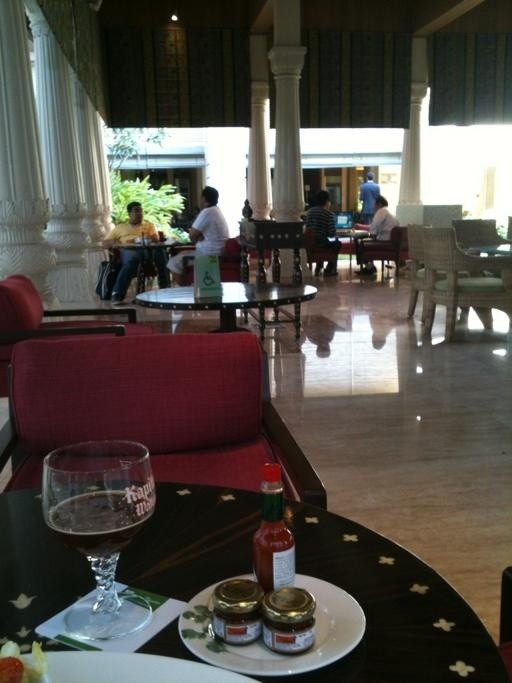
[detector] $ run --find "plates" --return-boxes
[176,571,370,678]
[1,651,261,683]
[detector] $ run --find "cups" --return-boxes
[41,440,156,641]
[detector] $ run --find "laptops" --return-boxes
[333,211,353,229]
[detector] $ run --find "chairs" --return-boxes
[407,224,492,328]
[451,216,512,244]
[302,226,407,271]
[422,226,512,340]
[0,332,328,511]
[497,566,512,683]
[0,274,155,360]
[170,235,271,286]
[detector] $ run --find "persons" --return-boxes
[165,185,229,286]
[359,171,381,225]
[101,201,170,309]
[305,190,341,277]
[354,195,400,274]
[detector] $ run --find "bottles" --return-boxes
[251,462,297,593]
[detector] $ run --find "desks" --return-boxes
[112,242,191,304]
[136,282,318,333]
[0,481,508,683]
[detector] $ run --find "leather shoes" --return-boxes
[354,267,377,274]
[324,269,338,276]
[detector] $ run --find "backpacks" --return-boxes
[96,261,114,300]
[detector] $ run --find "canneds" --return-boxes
[261,588,317,657]
[212,578,264,645]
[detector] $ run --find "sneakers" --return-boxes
[112,292,123,304]
